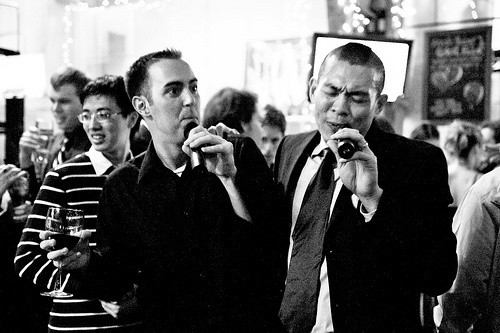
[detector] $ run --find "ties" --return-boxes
[278,148,337,333]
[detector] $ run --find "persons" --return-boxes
[1,42,500,333]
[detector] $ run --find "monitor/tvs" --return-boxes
[310,33,413,104]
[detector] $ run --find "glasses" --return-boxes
[76,111,120,123]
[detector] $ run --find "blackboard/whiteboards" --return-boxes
[422,24,493,127]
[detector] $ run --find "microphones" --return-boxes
[184,121,208,177]
[334,123,356,161]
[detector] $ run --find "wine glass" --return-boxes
[41,209,83,297]
[32,122,54,176]
